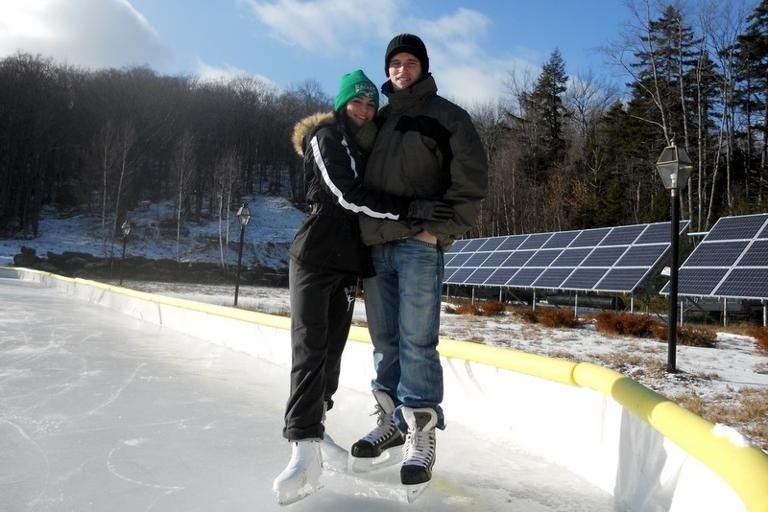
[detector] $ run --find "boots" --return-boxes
[391,403,439,485]
[349,385,406,458]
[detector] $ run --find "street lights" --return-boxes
[656,144,694,373]
[232,203,252,306]
[119,222,129,287]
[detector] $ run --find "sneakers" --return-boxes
[272,439,321,493]
[321,425,349,460]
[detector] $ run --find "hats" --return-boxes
[335,69,380,112]
[384,33,430,78]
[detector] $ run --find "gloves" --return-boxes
[406,197,456,223]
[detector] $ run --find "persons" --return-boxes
[351,33,490,486]
[272,66,455,490]
